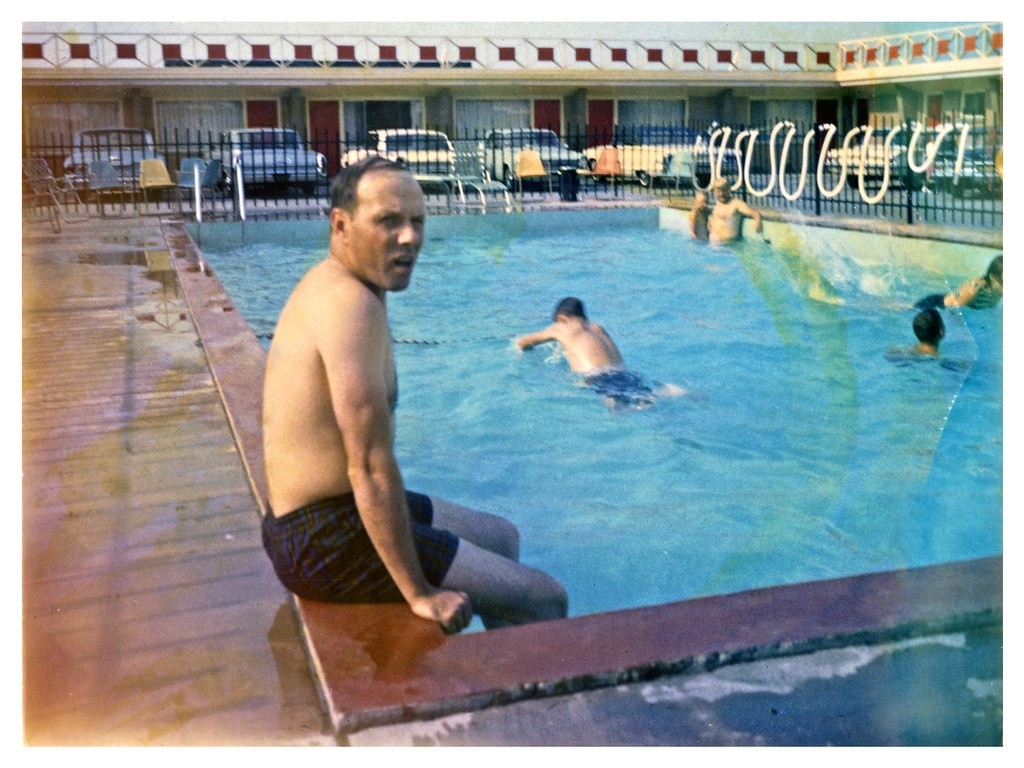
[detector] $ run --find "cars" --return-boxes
[821,125,949,196]
[64,127,166,200]
[583,126,745,187]
[205,129,329,197]
[934,125,1002,197]
[476,128,588,193]
[341,129,456,187]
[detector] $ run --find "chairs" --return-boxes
[511,151,553,201]
[448,149,512,205]
[200,159,229,218]
[139,158,177,215]
[22,157,90,231]
[88,160,126,219]
[589,149,622,199]
[179,158,208,215]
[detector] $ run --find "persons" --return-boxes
[516,296,626,374]
[913,309,946,354]
[915,254,1003,310]
[688,190,708,242]
[706,177,762,246]
[259,155,570,634]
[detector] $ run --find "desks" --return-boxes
[413,173,450,205]
[557,169,589,201]
